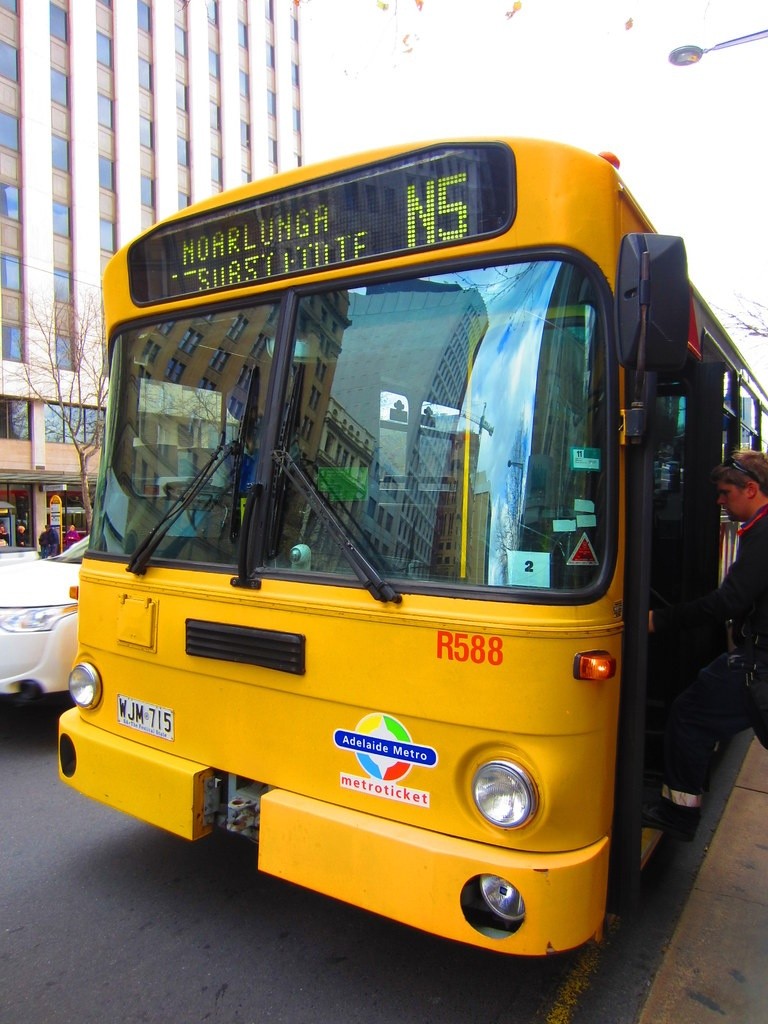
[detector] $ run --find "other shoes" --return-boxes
[638,800,698,841]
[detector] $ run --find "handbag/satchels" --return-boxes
[744,674,768,749]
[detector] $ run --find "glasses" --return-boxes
[724,457,759,483]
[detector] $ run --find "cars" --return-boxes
[0,533,91,696]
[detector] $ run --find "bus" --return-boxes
[55,134,768,958]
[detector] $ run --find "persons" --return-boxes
[64,523,79,551]
[641,450,768,841]
[39,523,58,559]
[0,524,27,547]
